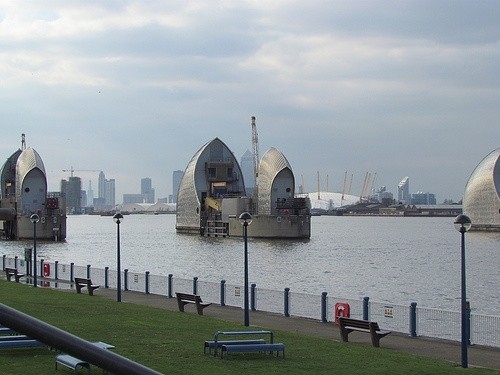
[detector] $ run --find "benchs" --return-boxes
[89,340,115,351]
[175,292,212,316]
[5,267,25,283]
[204,339,267,355]
[0,328,64,354]
[74,277,100,296]
[221,343,286,360]
[56,354,91,375]
[336,316,391,348]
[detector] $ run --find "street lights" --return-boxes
[113,213,124,302]
[30,214,40,287]
[239,212,253,326]
[453,214,472,369]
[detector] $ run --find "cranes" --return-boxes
[62,166,100,177]
[251,115,260,194]
